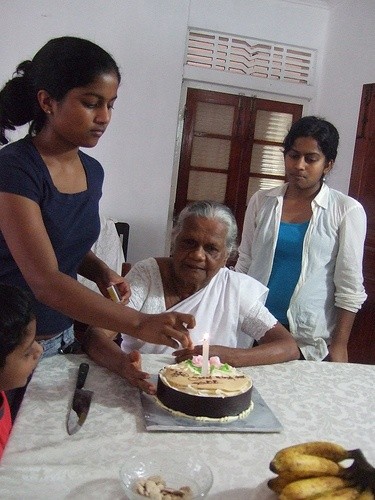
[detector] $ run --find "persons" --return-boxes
[83,205,302,395]
[236,116,371,361]
[0,283,44,464]
[0,37,196,425]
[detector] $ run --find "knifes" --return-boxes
[66,362,94,435]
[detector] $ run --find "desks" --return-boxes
[1,353,375,500]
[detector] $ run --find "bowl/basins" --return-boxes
[119,446,214,500]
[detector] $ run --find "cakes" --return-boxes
[155,355,254,422]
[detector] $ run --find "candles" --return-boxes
[202,332,209,376]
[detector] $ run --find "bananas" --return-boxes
[266,440,375,500]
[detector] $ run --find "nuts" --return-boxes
[133,472,192,500]
